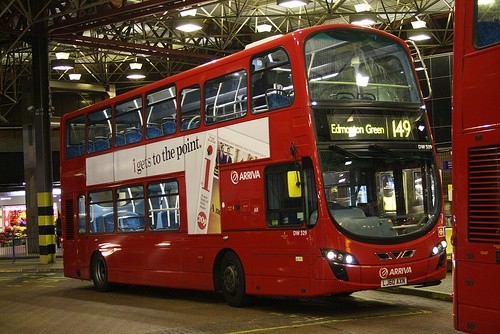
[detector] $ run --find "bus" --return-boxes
[448,0,500,334]
[62,22,449,308]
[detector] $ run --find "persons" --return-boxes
[54,214,62,249]
[213,144,232,177]
[19,218,27,226]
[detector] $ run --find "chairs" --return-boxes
[81,210,175,232]
[66,89,295,158]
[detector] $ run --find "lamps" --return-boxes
[176,8,202,32]
[51,52,81,80]
[277,0,432,42]
[257,22,272,33]
[126,57,146,80]
